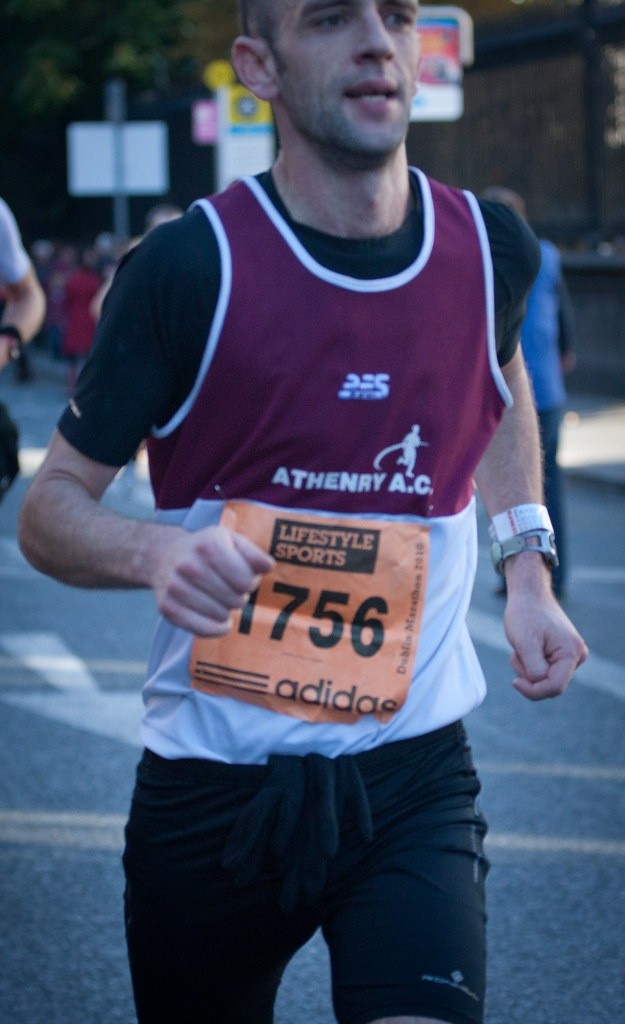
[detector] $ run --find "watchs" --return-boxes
[1,326,23,363]
[490,530,559,575]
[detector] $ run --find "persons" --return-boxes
[27,210,178,389]
[16,6,592,1024]
[484,185,576,603]
[0,197,48,513]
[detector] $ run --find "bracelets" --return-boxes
[487,504,555,544]
